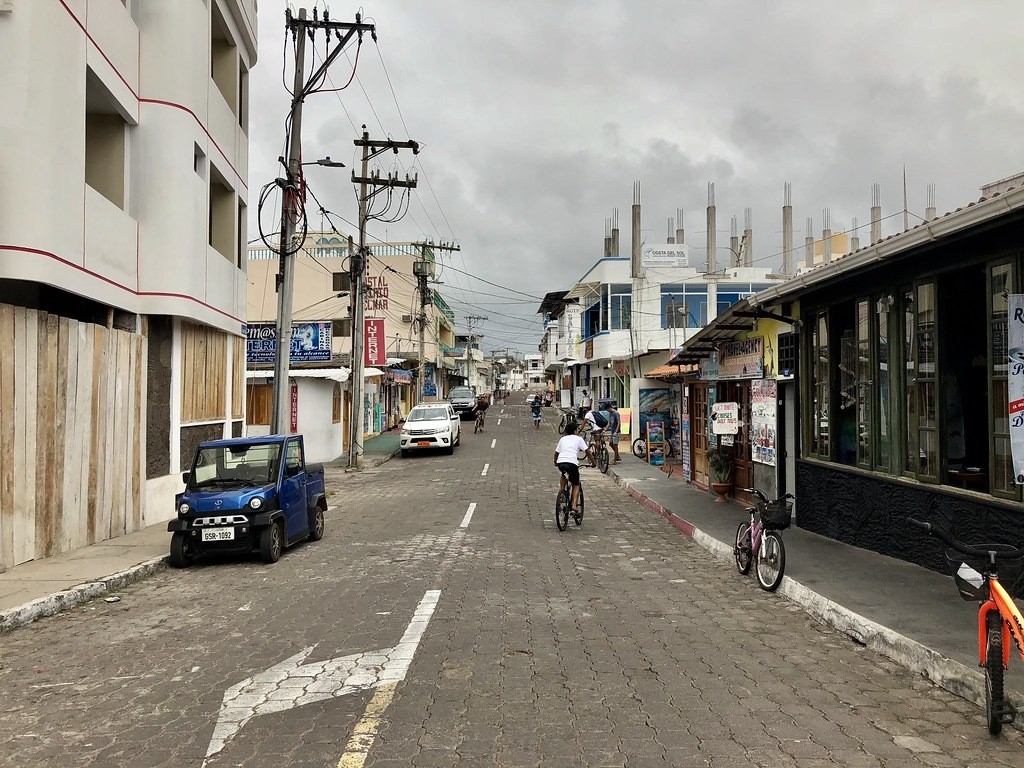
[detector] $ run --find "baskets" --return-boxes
[756,499,793,530]
[943,544,1024,600]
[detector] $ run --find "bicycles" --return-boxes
[555,463,595,531]
[558,408,609,474]
[734,489,796,589]
[472,410,485,435]
[906,517,1024,735]
[633,437,672,458]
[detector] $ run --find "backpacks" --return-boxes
[588,410,608,428]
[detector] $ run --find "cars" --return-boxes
[526,395,536,406]
[445,387,480,420]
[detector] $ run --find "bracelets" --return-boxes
[616,432,618,433]
[605,429,606,431]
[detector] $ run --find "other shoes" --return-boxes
[571,510,579,516]
[609,460,617,465]
[559,493,566,503]
[616,456,622,460]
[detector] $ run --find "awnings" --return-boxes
[563,281,600,310]
[447,373,468,386]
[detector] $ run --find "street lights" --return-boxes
[272,0,378,437]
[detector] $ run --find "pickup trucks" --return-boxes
[166,434,328,567]
[398,401,463,456]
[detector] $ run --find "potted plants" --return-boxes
[705,447,736,503]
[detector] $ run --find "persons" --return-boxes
[554,423,596,514]
[531,392,542,429]
[577,408,603,454]
[602,404,621,464]
[582,390,592,409]
[472,397,489,430]
[544,388,553,403]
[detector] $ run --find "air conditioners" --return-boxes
[402,315,411,322]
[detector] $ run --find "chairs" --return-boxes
[234,464,252,480]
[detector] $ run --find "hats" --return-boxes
[582,390,586,392]
